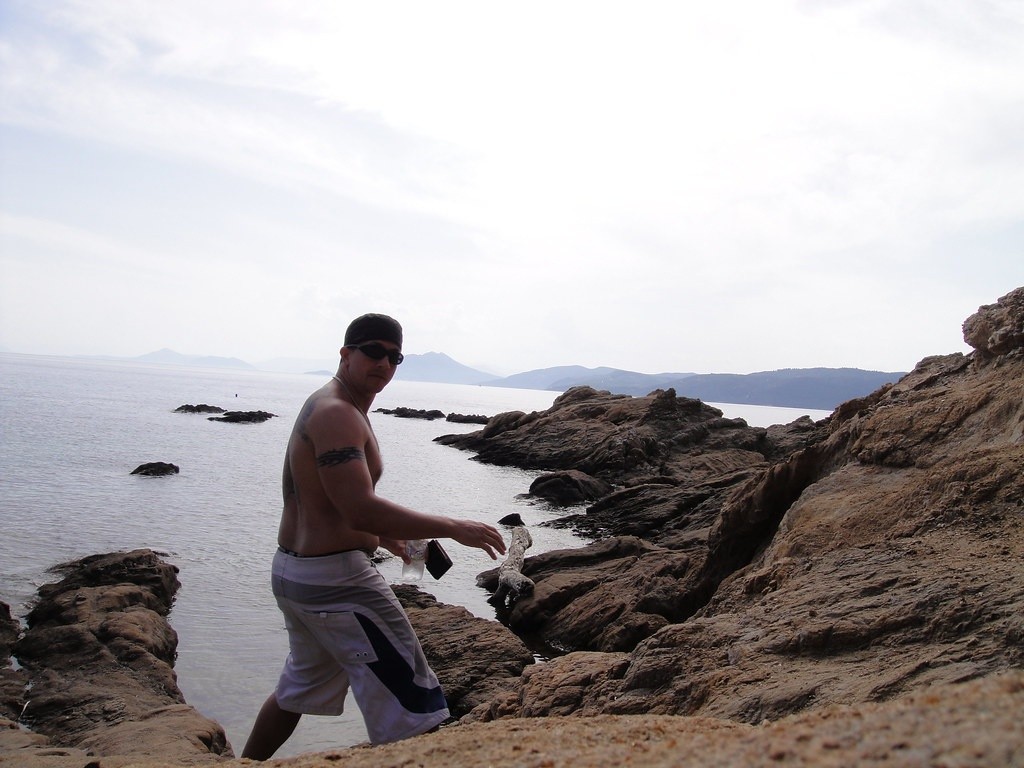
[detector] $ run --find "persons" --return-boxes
[241,311,508,766]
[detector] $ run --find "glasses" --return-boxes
[345,343,403,365]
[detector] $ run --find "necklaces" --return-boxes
[331,374,372,427]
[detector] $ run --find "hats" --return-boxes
[344,314,402,348]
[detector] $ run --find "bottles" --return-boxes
[402,539,427,581]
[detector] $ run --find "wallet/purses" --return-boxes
[423,540,453,580]
